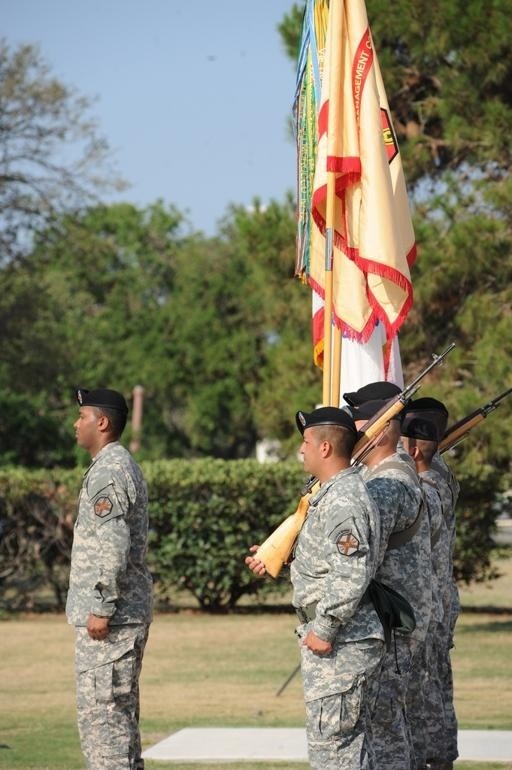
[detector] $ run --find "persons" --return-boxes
[63,384,156,770]
[240,406,387,770]
[340,380,461,770]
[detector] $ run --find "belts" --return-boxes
[295,601,318,625]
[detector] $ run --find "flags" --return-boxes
[291,0,419,386]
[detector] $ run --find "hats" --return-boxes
[343,381,405,406]
[296,407,358,440]
[400,416,439,442]
[400,397,449,420]
[75,388,129,414]
[342,399,402,421]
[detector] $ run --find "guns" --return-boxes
[438,388,512,454]
[250,343,456,582]
